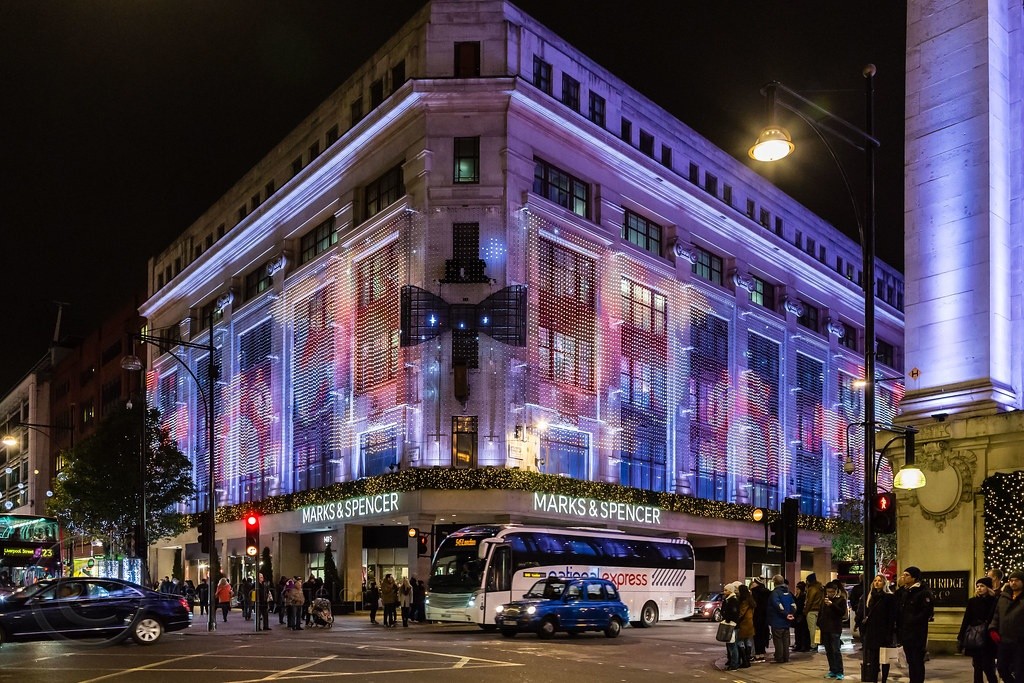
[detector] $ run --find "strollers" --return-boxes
[307,598,335,628]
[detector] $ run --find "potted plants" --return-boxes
[323,542,349,615]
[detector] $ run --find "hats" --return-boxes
[976,577,993,589]
[1008,569,1024,583]
[754,576,765,586]
[904,566,921,578]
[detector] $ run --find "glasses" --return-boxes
[260,577,264,578]
[723,589,728,592]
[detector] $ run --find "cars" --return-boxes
[0,577,194,646]
[495,577,630,638]
[685,592,729,622]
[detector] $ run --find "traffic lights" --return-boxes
[197,512,209,552]
[245,516,260,556]
[770,519,783,546]
[870,493,897,535]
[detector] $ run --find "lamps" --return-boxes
[930,413,948,423]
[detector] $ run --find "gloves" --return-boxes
[990,630,1001,645]
[956,642,964,653]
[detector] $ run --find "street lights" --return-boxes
[119,307,217,633]
[748,81,927,683]
[3,406,74,578]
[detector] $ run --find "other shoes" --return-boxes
[389,624,396,628]
[384,624,388,628]
[711,644,819,671]
[291,627,296,631]
[263,626,273,631]
[370,620,379,625]
[836,673,845,681]
[297,627,304,630]
[824,671,836,679]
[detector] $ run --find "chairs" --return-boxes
[73,585,82,593]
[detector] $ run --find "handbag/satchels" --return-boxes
[716,620,737,644]
[814,629,822,644]
[230,597,238,607]
[879,647,901,665]
[964,623,987,647]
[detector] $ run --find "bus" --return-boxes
[424,523,696,632]
[0,513,66,603]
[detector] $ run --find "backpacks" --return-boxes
[365,591,372,604]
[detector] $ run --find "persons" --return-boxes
[214,577,255,622]
[365,572,425,627]
[0,571,15,587]
[578,587,608,600]
[716,566,935,683]
[956,568,1024,683]
[11,527,53,542]
[460,564,476,582]
[59,586,73,599]
[20,576,38,586]
[259,570,332,630]
[146,576,209,615]
[544,586,559,599]
